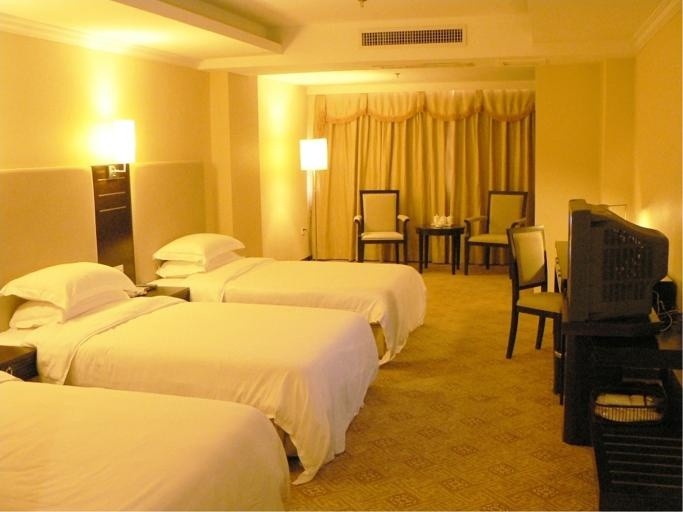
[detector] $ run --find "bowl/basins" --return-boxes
[446,217,456,224]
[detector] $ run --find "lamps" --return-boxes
[93,121,138,176]
[296,138,328,174]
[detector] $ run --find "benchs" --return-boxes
[585,371,681,512]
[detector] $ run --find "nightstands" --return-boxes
[130,279,192,303]
[0,343,40,381]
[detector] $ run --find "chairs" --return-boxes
[504,224,565,361]
[352,189,409,264]
[464,191,530,275]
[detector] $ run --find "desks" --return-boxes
[553,238,573,288]
[414,226,464,274]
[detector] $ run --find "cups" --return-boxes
[433,216,446,225]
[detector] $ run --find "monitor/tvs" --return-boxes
[566,198,669,322]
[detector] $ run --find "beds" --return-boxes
[145,234,426,369]
[0,261,382,487]
[0,371,292,511]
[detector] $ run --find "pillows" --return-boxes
[151,231,247,264]
[4,299,72,328]
[0,257,138,311]
[156,252,241,281]
